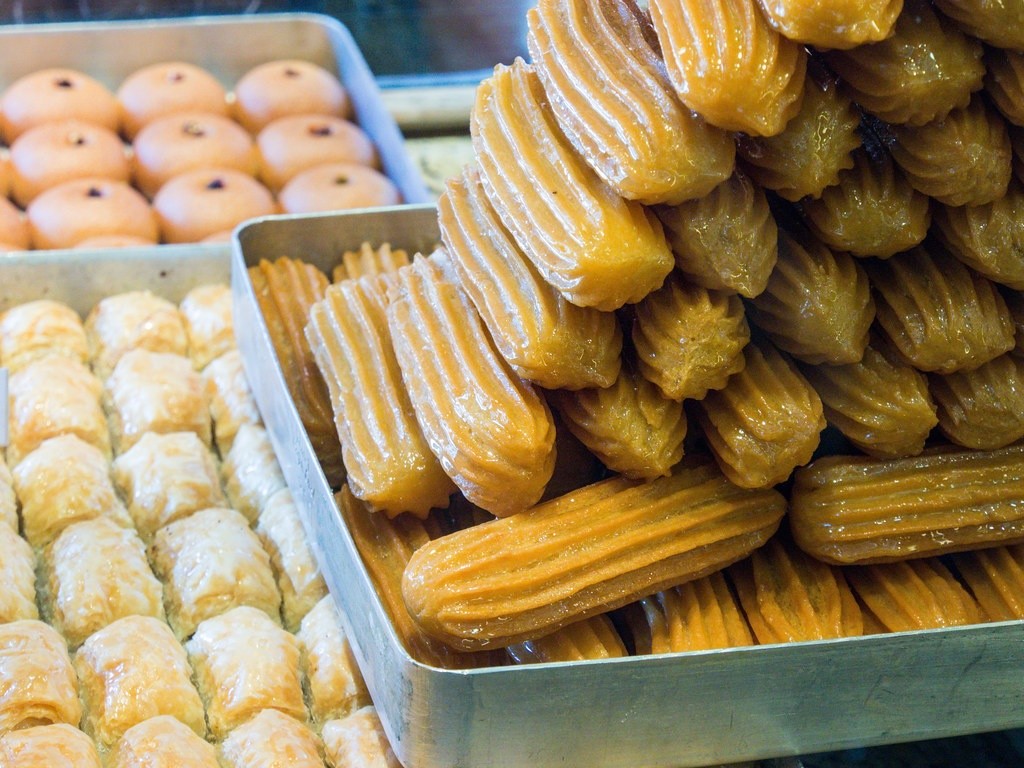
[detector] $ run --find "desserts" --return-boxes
[2,0,1024,766]
